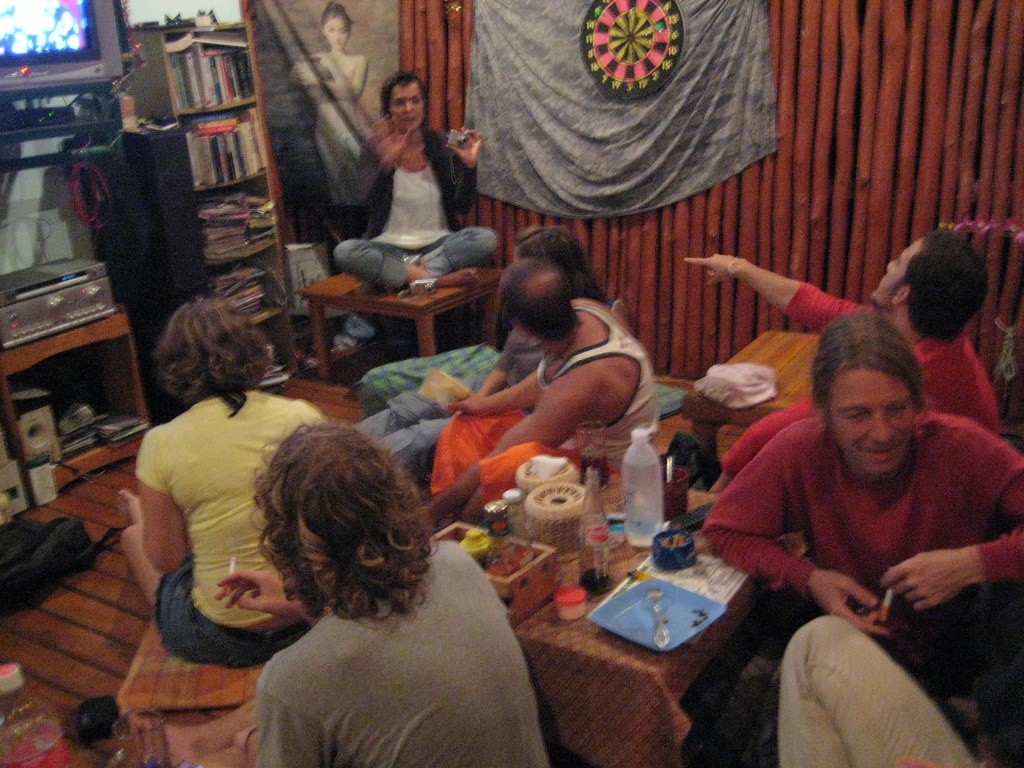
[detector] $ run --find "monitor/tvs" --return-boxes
[0,0,123,92]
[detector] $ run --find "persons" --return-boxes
[217,425,553,768]
[427,258,660,525]
[697,311,1024,768]
[116,298,331,666]
[331,70,499,296]
[289,1,376,204]
[457,223,609,416]
[681,230,1004,491]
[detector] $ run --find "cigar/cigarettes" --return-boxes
[228,557,236,575]
[880,587,895,622]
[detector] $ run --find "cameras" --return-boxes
[410,278,437,294]
[448,129,469,149]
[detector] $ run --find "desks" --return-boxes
[680,324,872,476]
[297,258,506,379]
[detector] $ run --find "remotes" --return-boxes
[668,502,716,532]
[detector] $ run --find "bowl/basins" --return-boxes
[418,367,471,415]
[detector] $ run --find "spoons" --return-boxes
[648,588,671,647]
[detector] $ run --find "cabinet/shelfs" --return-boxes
[69,120,306,389]
[129,19,264,114]
[0,313,158,500]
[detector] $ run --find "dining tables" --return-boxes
[491,435,748,768]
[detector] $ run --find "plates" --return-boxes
[589,577,727,653]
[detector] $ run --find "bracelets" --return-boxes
[728,259,743,274]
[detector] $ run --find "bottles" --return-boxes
[621,430,663,547]
[504,489,525,539]
[578,473,611,597]
[460,529,492,570]
[484,499,510,536]
[1,663,72,768]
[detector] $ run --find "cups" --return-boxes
[661,465,689,520]
[576,422,610,494]
[112,709,171,768]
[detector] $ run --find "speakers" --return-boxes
[14,389,61,464]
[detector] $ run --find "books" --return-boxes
[197,193,277,257]
[96,408,150,446]
[164,30,253,109]
[257,339,291,388]
[585,544,748,657]
[182,112,265,188]
[205,262,266,312]
[58,423,96,455]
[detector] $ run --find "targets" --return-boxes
[579,0,685,102]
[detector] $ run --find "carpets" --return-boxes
[118,625,297,706]
